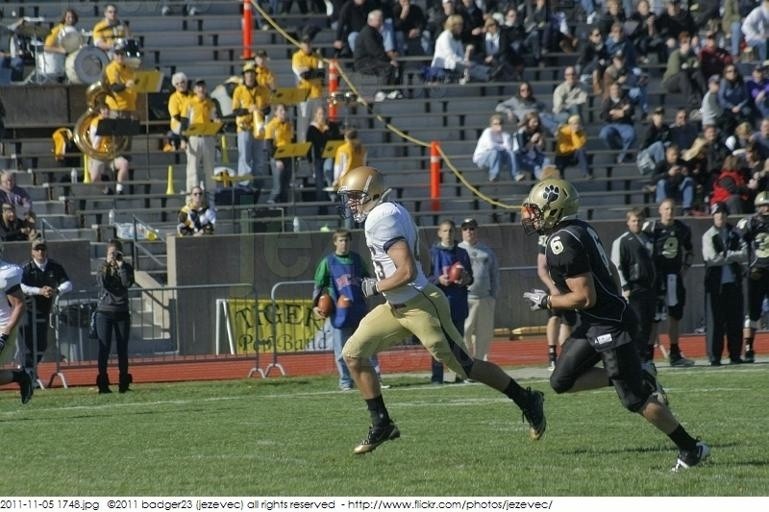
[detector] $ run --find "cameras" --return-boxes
[112,250,123,262]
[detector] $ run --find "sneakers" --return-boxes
[668,354,696,368]
[641,363,668,403]
[525,390,547,438]
[353,423,399,454]
[98,385,113,394]
[745,348,754,363]
[118,384,132,392]
[670,442,709,472]
[19,367,36,406]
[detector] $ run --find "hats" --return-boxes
[654,107,664,112]
[461,218,476,229]
[194,79,205,85]
[32,238,47,247]
[711,202,729,215]
[242,65,256,72]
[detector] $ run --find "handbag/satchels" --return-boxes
[89,270,119,338]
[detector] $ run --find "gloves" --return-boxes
[361,277,378,297]
[525,290,549,312]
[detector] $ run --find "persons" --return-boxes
[746,67,769,124]
[628,0,657,63]
[44,8,79,85]
[428,219,475,385]
[461,0,486,83]
[722,1,764,64]
[717,64,752,124]
[232,62,274,190]
[354,10,405,100]
[471,114,524,181]
[661,31,708,109]
[168,71,194,164]
[180,79,225,212]
[482,16,525,83]
[751,117,769,151]
[736,190,769,362]
[1,202,41,242]
[105,43,140,161]
[431,14,507,82]
[336,166,546,456]
[635,107,672,172]
[266,102,294,212]
[456,218,500,384]
[537,234,577,371]
[641,198,695,368]
[7,238,72,388]
[393,0,432,56]
[692,19,726,54]
[550,3,579,54]
[600,23,649,73]
[95,240,135,394]
[335,0,398,60]
[599,82,637,166]
[177,186,216,236]
[611,207,659,368]
[576,25,612,107]
[496,80,562,138]
[526,1,552,58]
[252,49,277,104]
[696,30,733,86]
[700,74,724,129]
[726,118,753,156]
[553,67,590,131]
[552,115,595,182]
[651,144,697,217]
[744,149,769,200]
[702,200,750,367]
[0,229,34,405]
[691,124,731,203]
[668,109,704,169]
[604,48,651,122]
[312,230,392,390]
[741,1,769,69]
[597,0,626,43]
[710,155,752,214]
[292,34,325,101]
[0,171,36,225]
[428,0,473,45]
[306,105,341,202]
[279,0,314,20]
[522,178,710,474]
[335,127,368,228]
[82,101,129,199]
[648,0,698,69]
[93,4,130,60]
[511,113,558,180]
[504,10,529,73]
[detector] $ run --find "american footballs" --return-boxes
[448,261,463,282]
[318,293,332,318]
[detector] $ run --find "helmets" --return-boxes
[337,167,385,220]
[754,190,769,206]
[523,180,580,234]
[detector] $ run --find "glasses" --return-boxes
[463,228,475,230]
[35,248,46,251]
[194,192,203,196]
[493,122,504,126]
[115,52,124,56]
